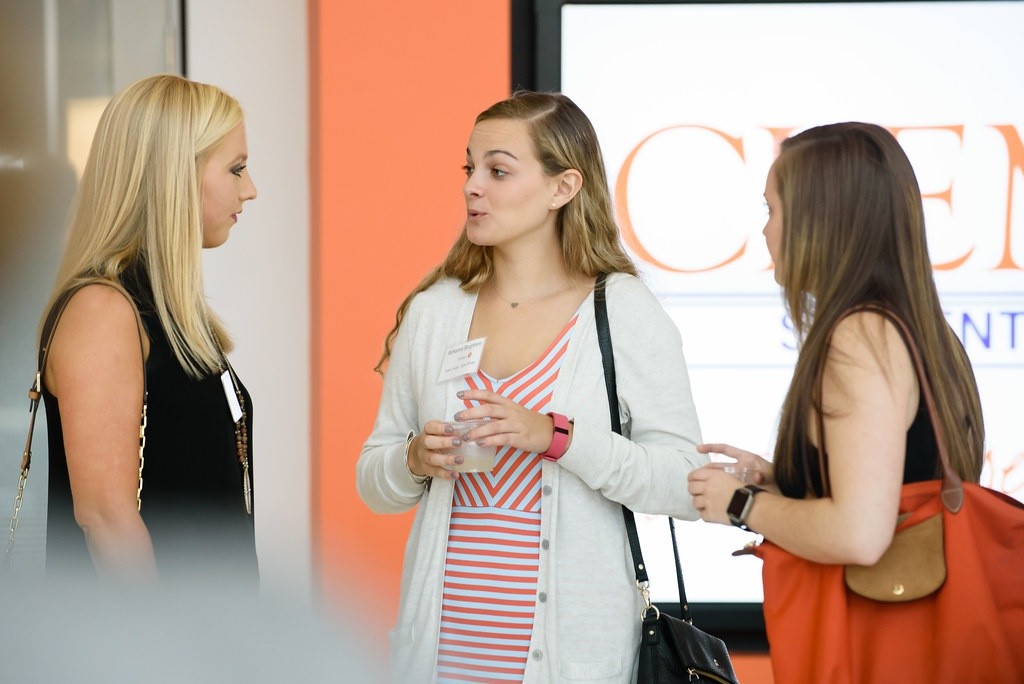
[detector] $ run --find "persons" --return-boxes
[355,90,712,684]
[38,76,260,596]
[687,124,985,684]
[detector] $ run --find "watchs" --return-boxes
[726,484,769,532]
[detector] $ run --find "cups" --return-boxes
[441,419,496,474]
[707,460,747,483]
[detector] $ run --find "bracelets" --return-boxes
[540,412,569,461]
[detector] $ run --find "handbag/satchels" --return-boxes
[731,302,1024,683]
[636,605,738,684]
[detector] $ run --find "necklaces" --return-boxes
[488,277,564,308]
[209,326,252,517]
